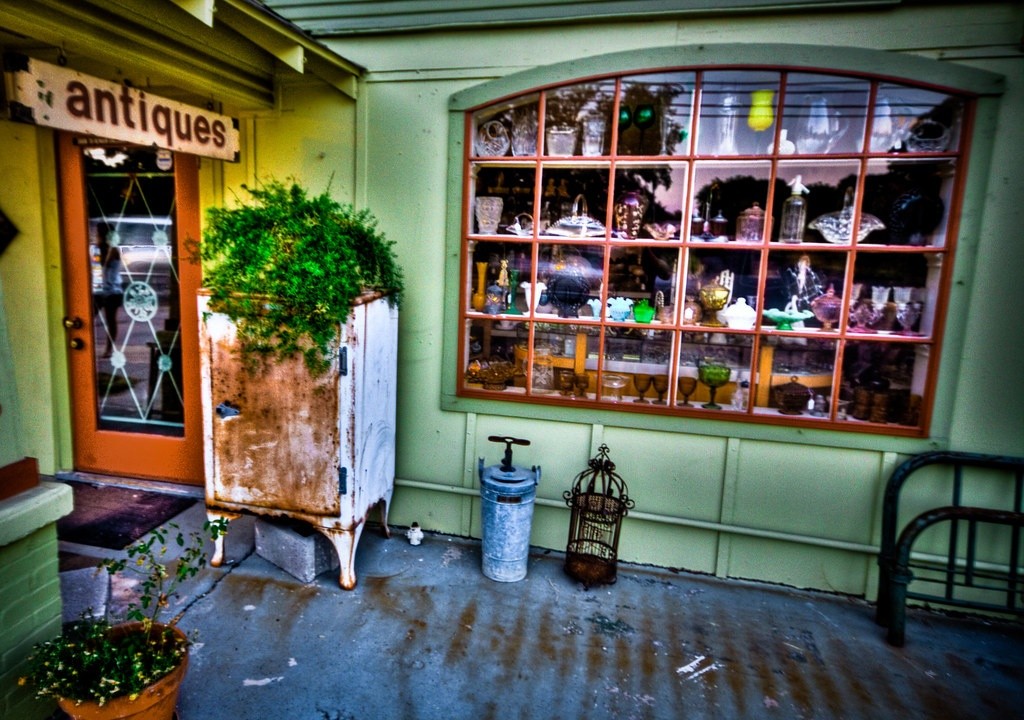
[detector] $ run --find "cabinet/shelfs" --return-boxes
[195,288,401,585]
[456,63,982,439]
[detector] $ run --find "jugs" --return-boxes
[858,93,913,152]
[796,95,848,153]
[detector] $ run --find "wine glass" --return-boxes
[657,111,670,156]
[698,363,730,409]
[633,375,651,404]
[575,373,589,397]
[619,104,655,155]
[847,285,926,336]
[677,377,697,408]
[652,375,669,404]
[748,89,773,155]
[559,373,573,395]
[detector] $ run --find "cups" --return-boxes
[523,358,554,394]
[512,110,536,156]
[546,126,576,156]
[602,374,629,402]
[475,197,504,234]
[582,121,605,156]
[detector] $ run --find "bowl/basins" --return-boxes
[774,376,810,415]
[723,283,841,332]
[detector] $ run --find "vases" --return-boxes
[58,622,189,720]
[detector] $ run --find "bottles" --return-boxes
[732,380,749,412]
[711,210,728,236]
[735,202,774,242]
[717,95,738,156]
[477,121,510,156]
[472,262,523,315]
[691,217,705,237]
[607,297,654,323]
[616,191,645,240]
[814,395,824,417]
[778,174,810,243]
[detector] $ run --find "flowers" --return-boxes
[16,511,228,708]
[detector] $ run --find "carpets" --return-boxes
[56,478,197,549]
